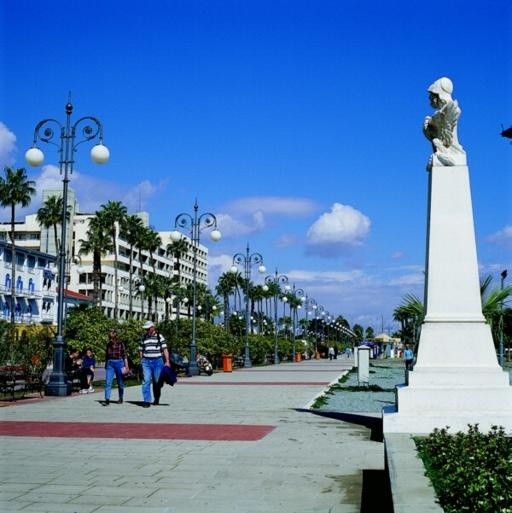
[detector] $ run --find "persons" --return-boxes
[82,347,96,384]
[345,345,350,358]
[334,344,338,358]
[327,343,335,360]
[101,330,129,406]
[422,75,468,172]
[403,343,414,370]
[137,320,172,408]
[64,347,96,394]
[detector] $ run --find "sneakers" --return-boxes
[101,399,109,406]
[142,400,159,408]
[117,398,123,404]
[78,386,95,394]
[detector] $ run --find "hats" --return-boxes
[141,320,155,330]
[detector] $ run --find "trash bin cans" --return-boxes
[295,352,301,362]
[316,352,320,359]
[222,352,232,372]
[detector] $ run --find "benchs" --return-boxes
[1,365,45,402]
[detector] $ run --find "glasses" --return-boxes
[145,327,152,330]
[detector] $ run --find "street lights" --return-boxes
[169,192,221,376]
[20,88,111,398]
[500,269,509,371]
[230,240,266,369]
[261,264,359,365]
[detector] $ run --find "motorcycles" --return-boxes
[194,351,214,377]
